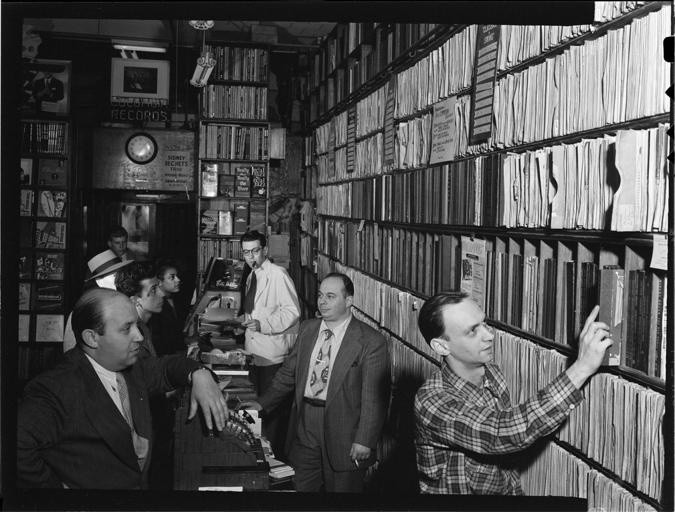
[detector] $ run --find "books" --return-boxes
[302,0,674,512]
[198,42,301,287]
[5,29,74,350]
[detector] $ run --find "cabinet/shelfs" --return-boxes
[302,1,673,512]
[17,119,85,407]
[194,42,272,301]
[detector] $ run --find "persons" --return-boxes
[234,230,302,461]
[63,250,133,354]
[114,263,200,421]
[150,263,198,355]
[0,287,230,488]
[234,271,391,493]
[106,226,138,280]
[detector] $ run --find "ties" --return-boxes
[115,373,149,472]
[308,329,335,399]
[242,272,257,314]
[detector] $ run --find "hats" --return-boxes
[83,248,135,282]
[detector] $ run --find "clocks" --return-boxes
[124,132,158,165]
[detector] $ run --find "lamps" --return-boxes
[188,19,217,88]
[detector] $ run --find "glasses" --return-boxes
[243,248,260,256]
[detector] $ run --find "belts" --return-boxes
[304,398,326,407]
